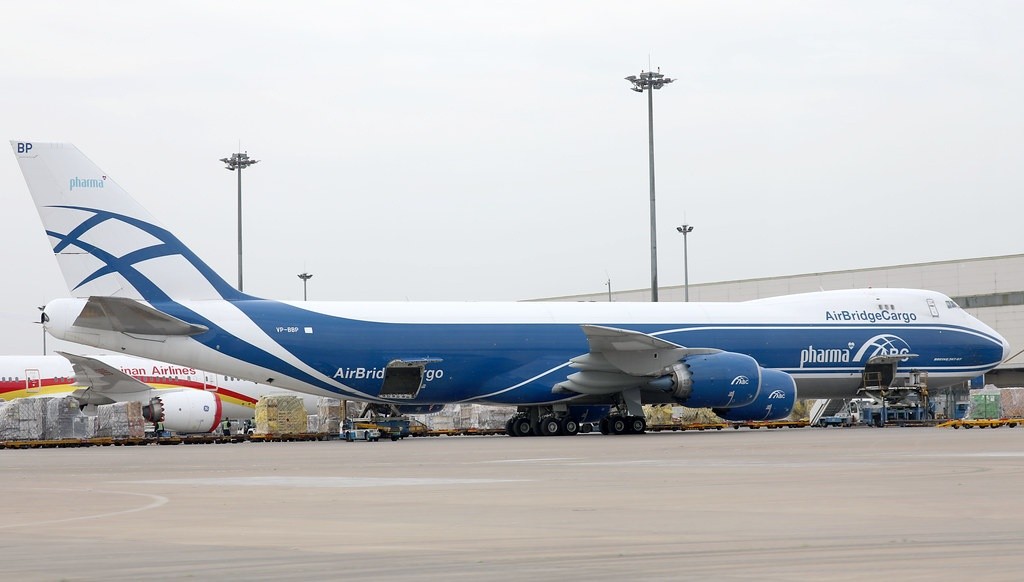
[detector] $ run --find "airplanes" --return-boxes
[0,137,1005,446]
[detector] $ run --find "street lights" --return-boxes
[219,150,261,292]
[624,70,672,301]
[676,224,694,302]
[297,272,313,301]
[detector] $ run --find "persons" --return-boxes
[222,417,231,442]
[155,418,164,444]
[248,417,256,428]
